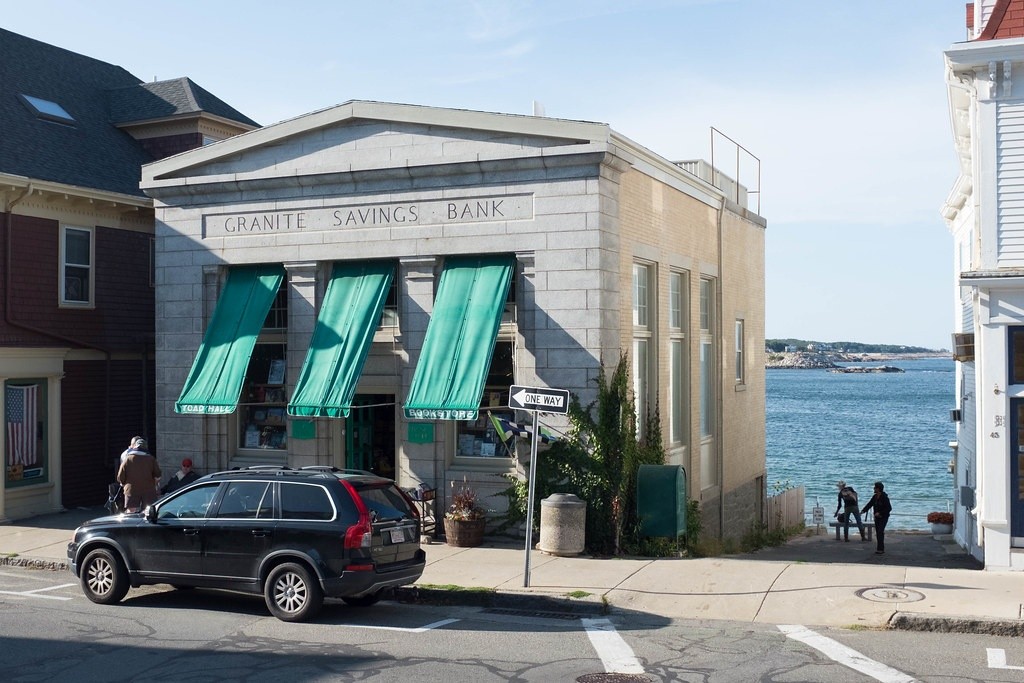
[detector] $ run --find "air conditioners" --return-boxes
[950,409,960,421]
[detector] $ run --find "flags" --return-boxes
[7,386,39,466]
[489,415,561,445]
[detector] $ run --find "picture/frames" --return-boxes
[268,358,287,384]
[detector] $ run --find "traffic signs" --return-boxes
[506,383,572,416]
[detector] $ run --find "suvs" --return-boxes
[67,465,426,628]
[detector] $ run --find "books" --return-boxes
[458,392,516,458]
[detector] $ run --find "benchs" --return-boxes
[830,521,876,541]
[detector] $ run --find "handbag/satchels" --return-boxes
[837,512,852,523]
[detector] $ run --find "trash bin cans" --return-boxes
[636,464,687,549]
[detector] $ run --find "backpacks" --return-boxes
[840,488,858,507]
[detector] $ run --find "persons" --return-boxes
[860,483,892,553]
[834,480,869,542]
[117,437,204,509]
[370,448,391,476]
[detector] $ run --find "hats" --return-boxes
[836,481,847,486]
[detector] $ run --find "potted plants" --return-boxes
[442,473,497,547]
[927,512,954,534]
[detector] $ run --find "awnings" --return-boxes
[173,256,518,422]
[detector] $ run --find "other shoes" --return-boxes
[844,539,850,542]
[862,537,868,541]
[875,551,885,554]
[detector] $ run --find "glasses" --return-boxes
[183,465,191,468]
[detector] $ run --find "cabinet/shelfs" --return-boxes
[411,488,438,535]
[243,384,286,448]
